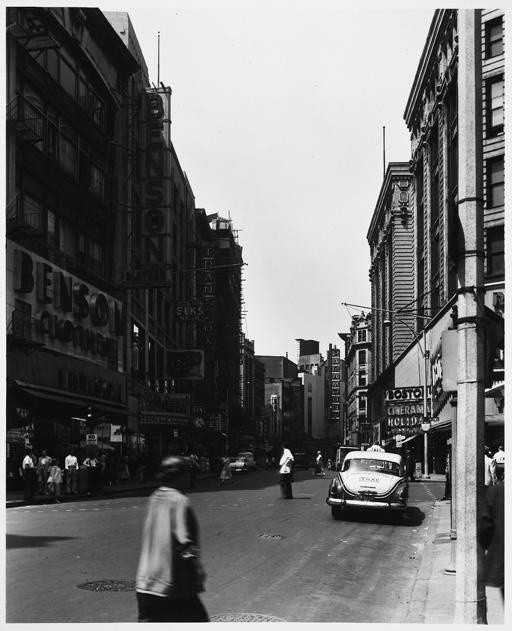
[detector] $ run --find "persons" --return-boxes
[264,454,282,472]
[313,450,326,477]
[135,457,210,622]
[179,446,235,489]
[278,447,296,499]
[20,447,110,502]
[446,441,504,624]
[405,449,416,482]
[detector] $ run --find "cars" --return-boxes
[327,444,409,522]
[294,451,308,471]
[328,459,334,471]
[227,450,257,474]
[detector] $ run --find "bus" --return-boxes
[336,445,360,471]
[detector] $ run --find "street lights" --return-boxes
[225,380,251,458]
[382,315,430,479]
[67,405,105,496]
